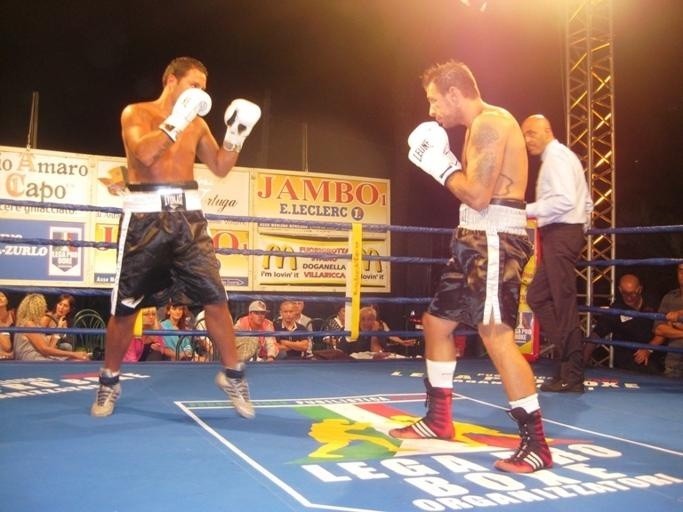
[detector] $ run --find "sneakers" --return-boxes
[213,364,256,421]
[539,374,585,394]
[88,365,124,419]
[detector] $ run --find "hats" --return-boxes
[248,299,270,313]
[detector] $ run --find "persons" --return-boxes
[519,114,596,396]
[0,290,88,362]
[387,60,552,477]
[89,55,261,422]
[583,262,683,377]
[122,300,387,361]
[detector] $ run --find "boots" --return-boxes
[491,404,554,475]
[388,378,458,441]
